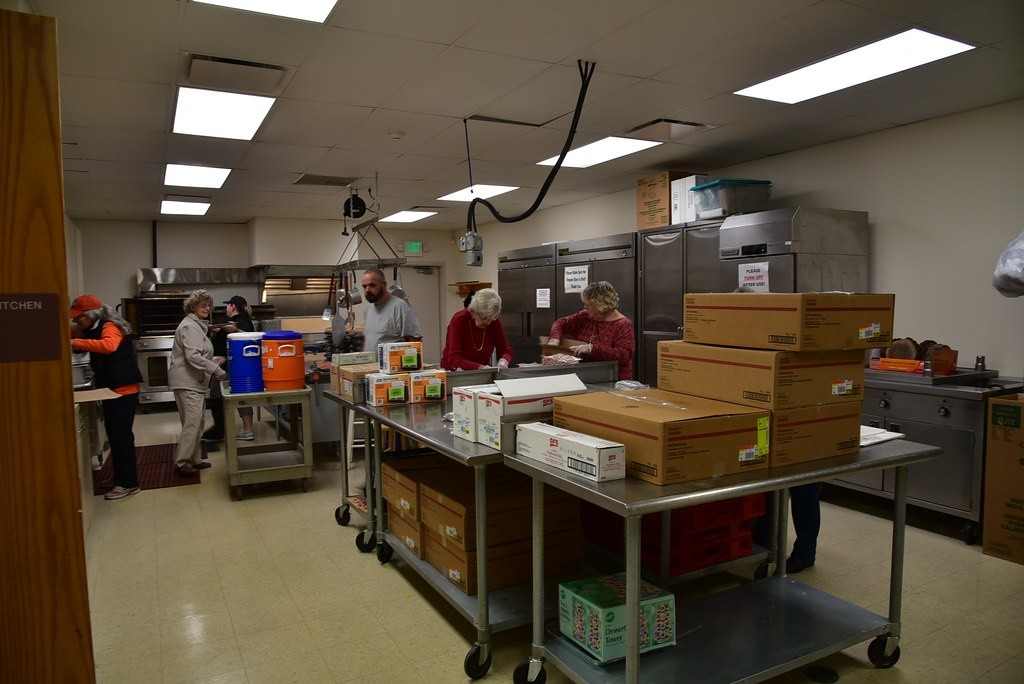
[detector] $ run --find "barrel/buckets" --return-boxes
[261,330,305,390]
[226,332,264,394]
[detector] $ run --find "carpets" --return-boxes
[94,438,208,496]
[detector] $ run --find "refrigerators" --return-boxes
[497,243,556,368]
[556,232,636,379]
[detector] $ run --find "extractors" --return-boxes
[114,293,216,335]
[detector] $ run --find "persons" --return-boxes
[222,295,256,439]
[547,280,633,379]
[69,295,144,499]
[361,269,424,506]
[439,288,513,371]
[786,483,821,574]
[168,290,229,476]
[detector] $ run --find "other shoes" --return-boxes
[192,462,211,469]
[174,464,197,476]
[786,555,815,573]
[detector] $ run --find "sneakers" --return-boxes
[105,486,140,499]
[101,478,121,487]
[236,429,255,439]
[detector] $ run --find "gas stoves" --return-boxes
[135,335,175,351]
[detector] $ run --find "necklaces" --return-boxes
[590,314,613,343]
[468,319,486,352]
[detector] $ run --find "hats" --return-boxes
[222,295,248,310]
[69,295,102,318]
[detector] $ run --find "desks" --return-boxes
[504,438,945,684]
[322,382,781,681]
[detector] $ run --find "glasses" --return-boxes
[477,313,487,321]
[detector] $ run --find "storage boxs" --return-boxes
[683,291,895,353]
[552,388,772,486]
[539,335,588,356]
[769,401,863,468]
[588,493,766,538]
[656,340,865,411]
[418,470,581,553]
[423,526,585,596]
[516,421,627,484]
[636,171,774,231]
[983,393,1024,566]
[475,373,588,452]
[387,501,425,561]
[558,572,677,663]
[452,384,499,443]
[380,454,507,523]
[329,342,448,408]
[580,513,754,577]
